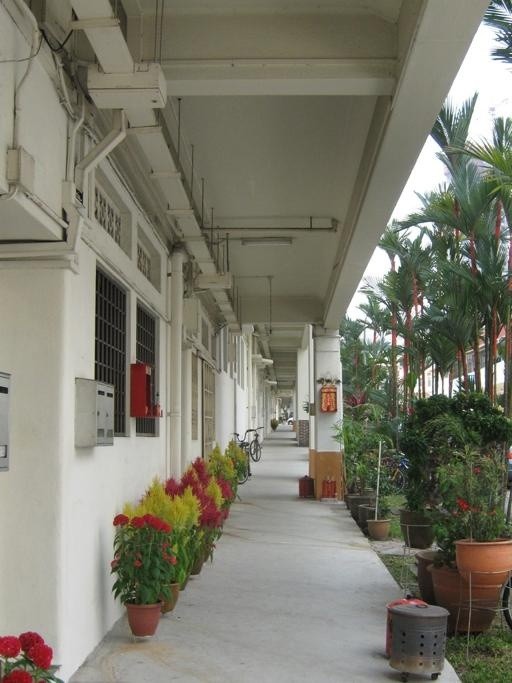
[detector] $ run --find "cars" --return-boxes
[476,446,512,483]
[287,417,294,425]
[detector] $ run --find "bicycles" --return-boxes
[385,453,415,492]
[228,427,257,485]
[250,426,266,461]
[503,575,512,632]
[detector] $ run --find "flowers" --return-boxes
[106,435,249,607]
[0,630,65,682]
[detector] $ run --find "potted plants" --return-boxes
[330,392,511,633]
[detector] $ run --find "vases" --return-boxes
[158,582,180,613]
[122,598,166,638]
